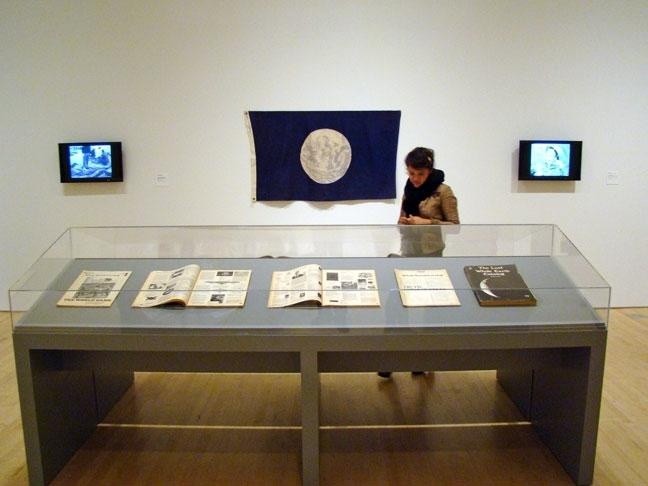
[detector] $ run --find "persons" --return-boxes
[375,146,460,380]
[533,144,568,177]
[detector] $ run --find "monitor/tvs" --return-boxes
[518,140,582,181]
[58,142,123,182]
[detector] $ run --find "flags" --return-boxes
[242,108,402,202]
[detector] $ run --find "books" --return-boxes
[465,264,538,307]
[394,267,462,308]
[129,264,253,308]
[56,269,132,307]
[266,263,382,309]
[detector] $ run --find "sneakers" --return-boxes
[377,373,393,379]
[411,371,425,377]
